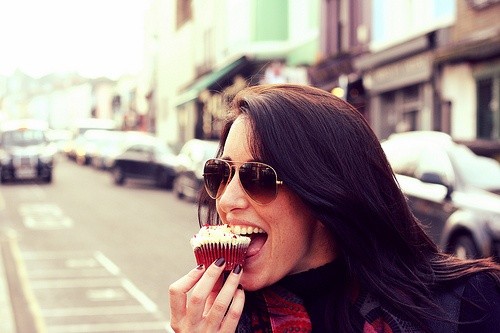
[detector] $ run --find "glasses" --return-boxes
[202,159,285,206]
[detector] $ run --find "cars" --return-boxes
[0,121,52,182]
[112,141,174,189]
[378,130,499,261]
[65,130,146,171]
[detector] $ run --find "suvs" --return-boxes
[174,139,223,203]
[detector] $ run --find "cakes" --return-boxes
[190,224,251,271]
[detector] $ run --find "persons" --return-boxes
[169,83,500,333]
